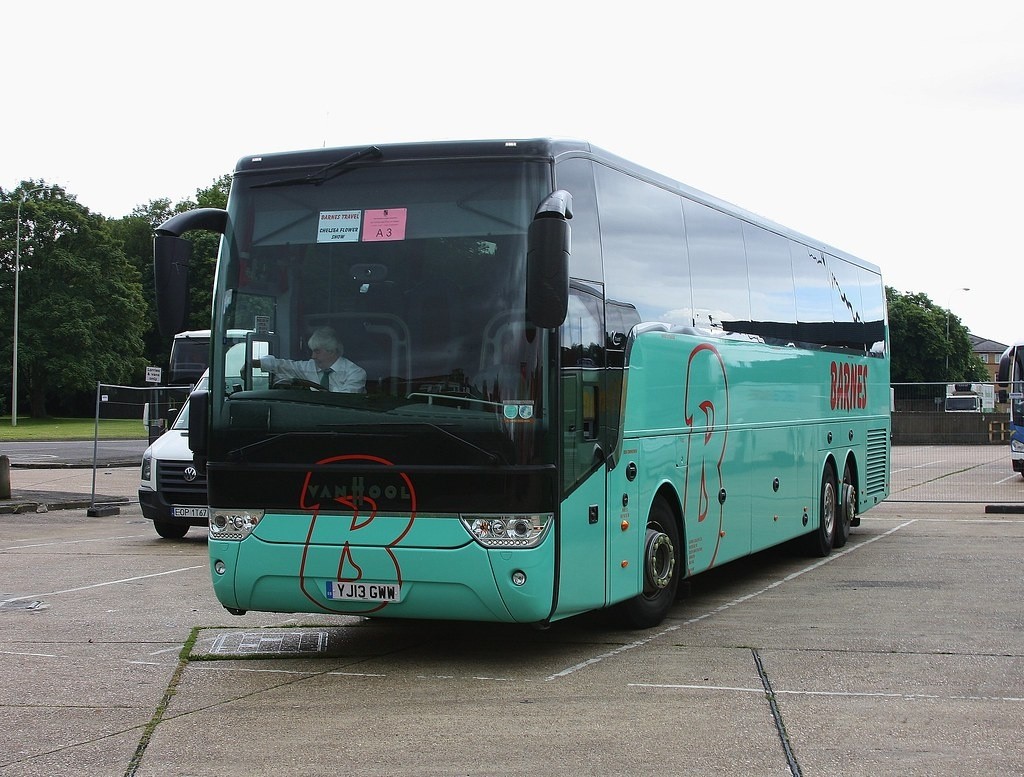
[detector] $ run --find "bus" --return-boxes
[996,341,1024,478]
[165,327,273,430]
[152,138,895,631]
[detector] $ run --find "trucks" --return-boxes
[945,384,995,413]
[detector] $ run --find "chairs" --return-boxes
[341,334,385,395]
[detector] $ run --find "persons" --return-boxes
[239,326,368,393]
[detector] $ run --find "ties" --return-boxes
[320,368,333,391]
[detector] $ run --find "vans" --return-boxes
[137,342,270,539]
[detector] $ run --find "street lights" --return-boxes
[10,187,69,426]
[946,287,970,370]
